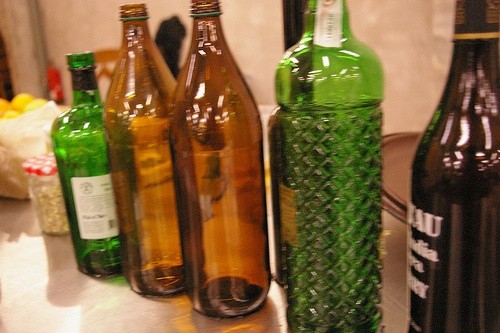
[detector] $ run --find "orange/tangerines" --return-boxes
[0,93,49,121]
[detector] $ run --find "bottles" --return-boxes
[403,0,500,332]
[103,3,207,297]
[266,0,384,333]
[51,50,150,282]
[168,1,269,319]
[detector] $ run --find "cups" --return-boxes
[21,151,72,238]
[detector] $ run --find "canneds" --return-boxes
[22,151,71,237]
